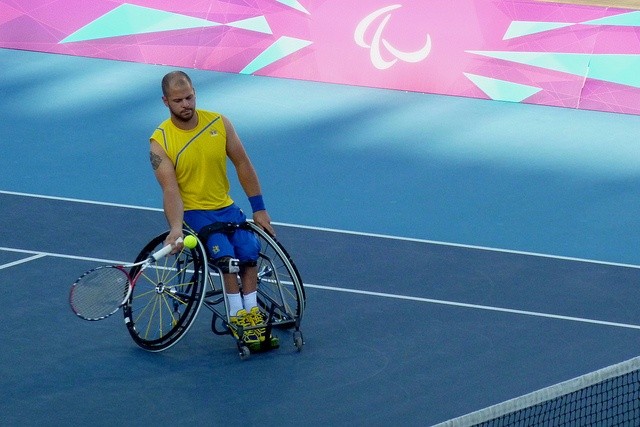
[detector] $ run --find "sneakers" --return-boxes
[230,309,260,348]
[251,307,279,348]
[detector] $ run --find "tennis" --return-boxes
[183,234,197,249]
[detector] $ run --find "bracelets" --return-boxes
[248,195,267,212]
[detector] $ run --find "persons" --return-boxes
[150,71,280,351]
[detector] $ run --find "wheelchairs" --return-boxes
[123,218,307,359]
